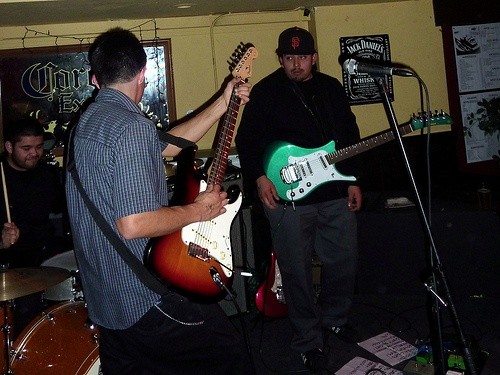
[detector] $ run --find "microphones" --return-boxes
[344,59,414,77]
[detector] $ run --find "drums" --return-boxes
[8,300,103,375]
[0,297,16,375]
[41,249,84,301]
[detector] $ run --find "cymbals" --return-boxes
[0,266,69,302]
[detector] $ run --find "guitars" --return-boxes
[254,249,289,318]
[263,109,455,202]
[144,45,260,304]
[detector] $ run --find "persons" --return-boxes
[236,27,363,371]
[65,27,252,375]
[0,117,69,329]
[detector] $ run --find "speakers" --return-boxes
[428,209,500,300]
[217,207,256,316]
[353,205,428,295]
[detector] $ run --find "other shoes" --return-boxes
[329,323,359,342]
[301,347,327,369]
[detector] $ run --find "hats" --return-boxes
[278,27,314,56]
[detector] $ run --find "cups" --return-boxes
[477,181,491,209]
